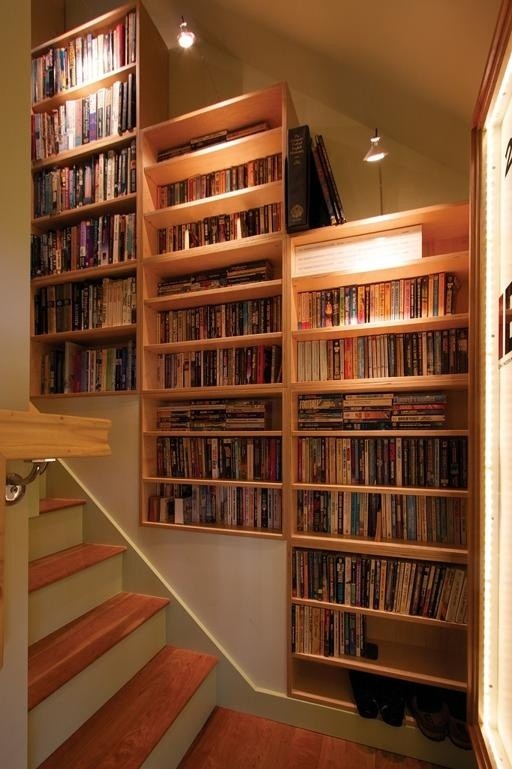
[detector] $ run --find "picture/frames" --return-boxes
[466,1,512,769]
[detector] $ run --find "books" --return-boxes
[31,10,137,393]
[147,123,282,533]
[285,125,347,231]
[289,271,468,664]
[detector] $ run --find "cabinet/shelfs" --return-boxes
[138,79,299,542]
[30,0,169,400]
[287,202,471,729]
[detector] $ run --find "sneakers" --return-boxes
[448,709,475,752]
[412,692,447,742]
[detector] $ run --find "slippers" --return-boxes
[351,668,378,718]
[383,682,406,730]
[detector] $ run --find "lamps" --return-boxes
[178,15,222,102]
[364,126,389,215]
